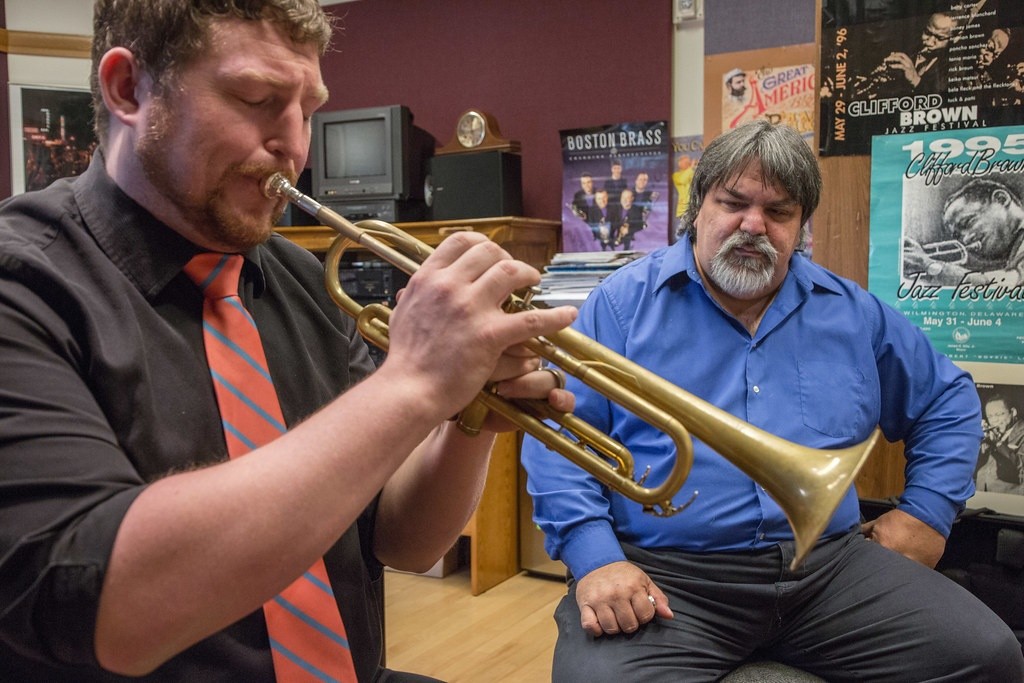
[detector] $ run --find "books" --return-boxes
[531,250,640,308]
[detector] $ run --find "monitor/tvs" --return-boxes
[310,104,436,202]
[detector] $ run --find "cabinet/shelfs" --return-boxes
[270,218,563,596]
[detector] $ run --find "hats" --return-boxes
[725,68,746,84]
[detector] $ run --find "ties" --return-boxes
[183,253,362,683]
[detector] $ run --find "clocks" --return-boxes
[434,107,522,156]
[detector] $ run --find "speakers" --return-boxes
[431,152,524,220]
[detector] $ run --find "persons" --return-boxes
[975,24,1022,105]
[672,155,698,217]
[573,160,654,252]
[0,0,579,683]
[520,120,1024,683]
[975,393,1024,493]
[853,10,957,109]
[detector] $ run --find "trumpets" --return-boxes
[903,240,982,278]
[258,169,884,570]
